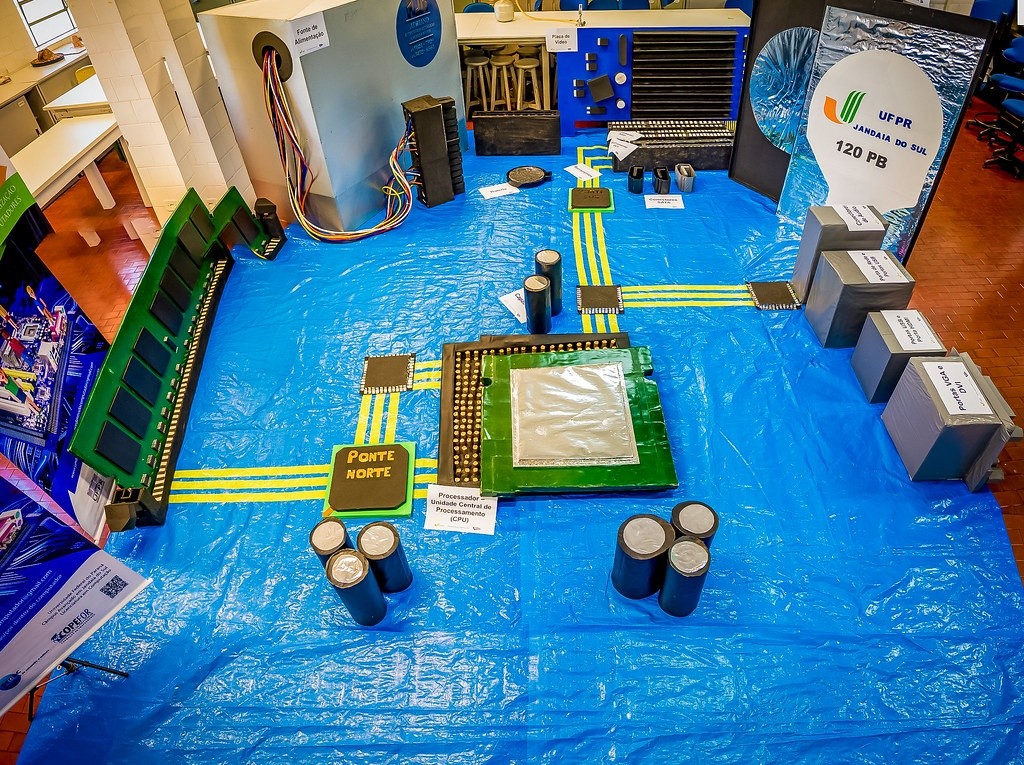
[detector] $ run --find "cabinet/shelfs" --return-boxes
[0,44,114,212]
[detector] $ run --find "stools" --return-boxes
[464,44,541,122]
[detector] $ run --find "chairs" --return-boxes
[74,62,96,84]
[965,36,1024,180]
[463,0,753,19]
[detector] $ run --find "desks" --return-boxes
[9,113,152,208]
[455,9,751,110]
[42,73,112,120]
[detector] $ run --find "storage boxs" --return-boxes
[790,206,1024,493]
